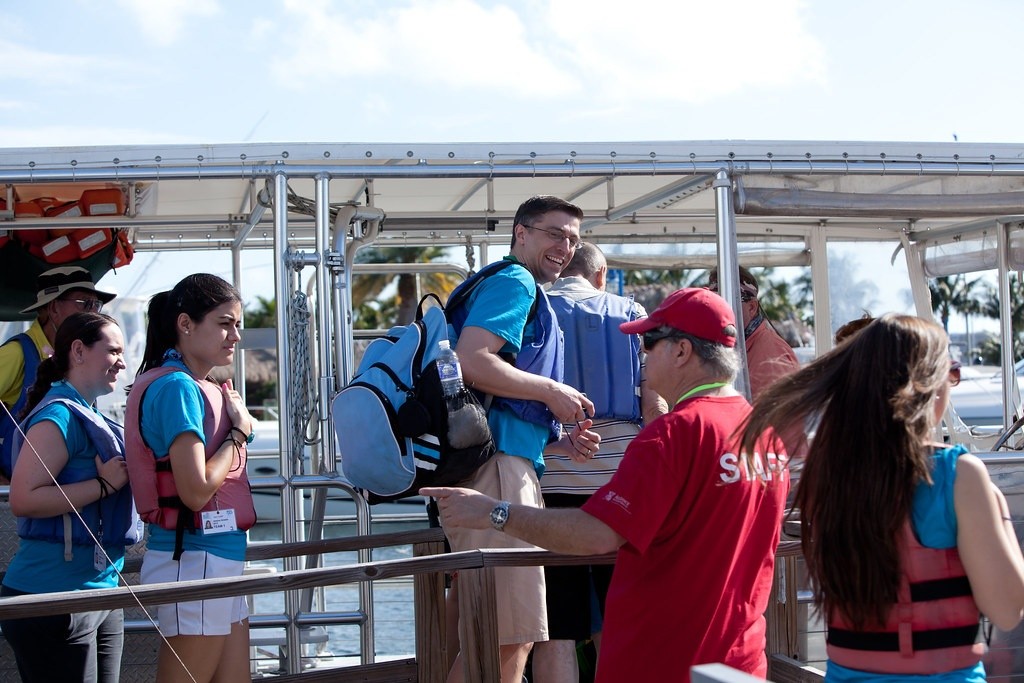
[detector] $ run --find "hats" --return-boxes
[620,288,737,350]
[19,267,117,315]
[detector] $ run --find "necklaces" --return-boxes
[676,382,729,403]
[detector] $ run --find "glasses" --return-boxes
[948,361,961,387]
[638,330,678,355]
[522,224,585,249]
[56,296,103,316]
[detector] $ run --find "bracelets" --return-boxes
[223,425,255,472]
[1001,516,1012,520]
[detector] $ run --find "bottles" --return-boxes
[436,340,464,395]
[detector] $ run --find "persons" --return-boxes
[1,266,116,495]
[420,285,790,683]
[125,273,256,683]
[444,197,601,683]
[730,315,1024,683]
[1,313,132,683]
[522,241,667,683]
[205,520,213,529]
[707,266,810,490]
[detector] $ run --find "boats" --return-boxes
[791,344,1024,430]
[237,406,430,525]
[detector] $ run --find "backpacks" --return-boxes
[331,258,539,505]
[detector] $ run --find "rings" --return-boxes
[585,449,591,457]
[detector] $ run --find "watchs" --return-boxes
[490,501,512,532]
